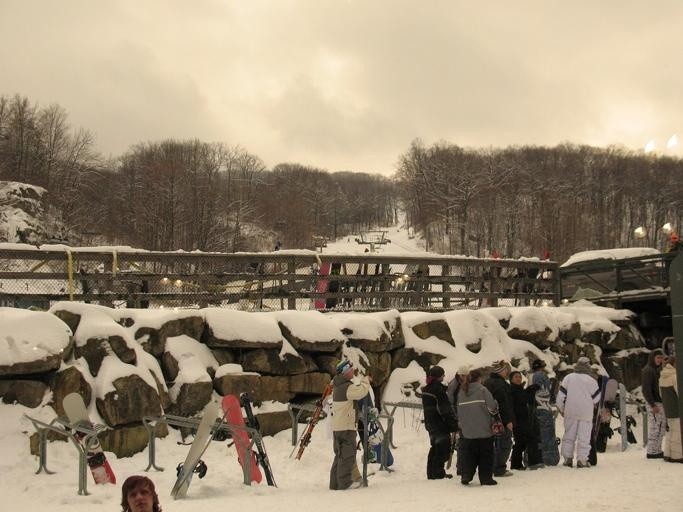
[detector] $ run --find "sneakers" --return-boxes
[494,462,543,476]
[462,477,498,486]
[564,458,591,467]
[346,481,361,490]
[646,451,683,463]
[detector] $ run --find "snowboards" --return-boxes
[353,385,394,466]
[63,393,116,485]
[171,401,218,499]
[296,378,334,460]
[240,392,276,486]
[590,375,628,453]
[524,388,538,469]
[222,395,262,483]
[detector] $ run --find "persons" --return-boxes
[119,474,162,512]
[421,347,683,484]
[329,359,374,491]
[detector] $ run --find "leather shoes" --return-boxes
[444,473,453,479]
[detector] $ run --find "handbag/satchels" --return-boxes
[492,420,504,434]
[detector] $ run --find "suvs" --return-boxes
[534,246,664,300]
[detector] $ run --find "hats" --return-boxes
[458,365,469,375]
[337,360,353,376]
[495,363,507,373]
[430,366,444,377]
[574,356,591,372]
[532,358,547,369]
